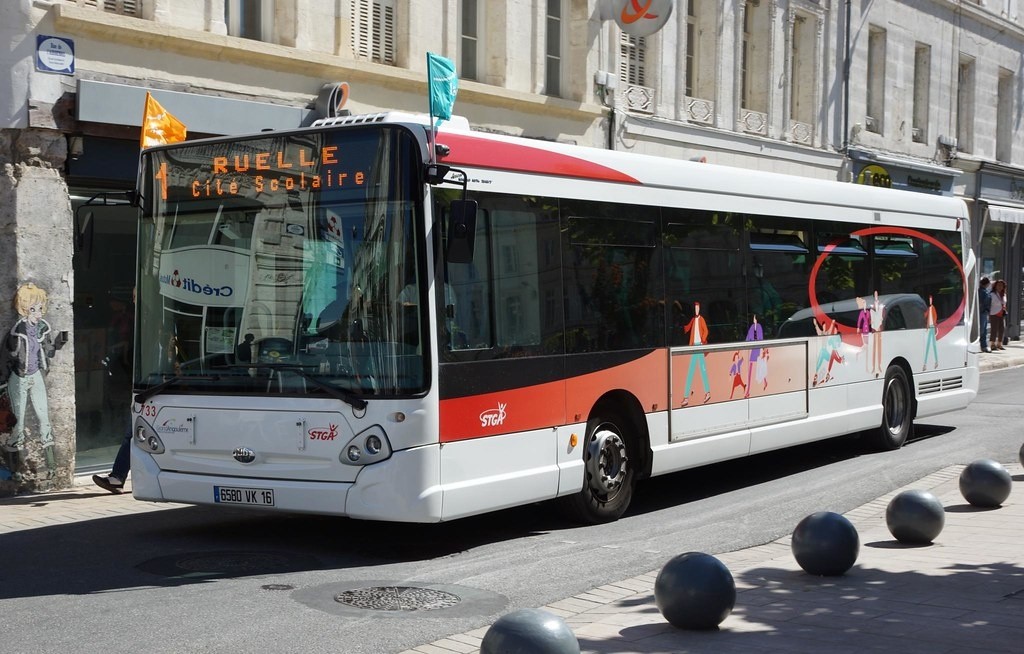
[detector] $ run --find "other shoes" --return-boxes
[981,346,990,353]
[990,344,1005,351]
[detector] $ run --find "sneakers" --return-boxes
[92,474,125,496]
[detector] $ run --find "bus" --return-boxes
[129,110,980,524]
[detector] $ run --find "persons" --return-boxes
[147,325,181,384]
[391,279,457,334]
[238,334,255,376]
[987,279,1008,350]
[349,334,378,396]
[93,284,136,495]
[976,277,993,353]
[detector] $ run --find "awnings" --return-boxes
[978,198,1024,246]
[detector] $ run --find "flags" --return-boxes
[427,51,458,126]
[140,90,188,150]
